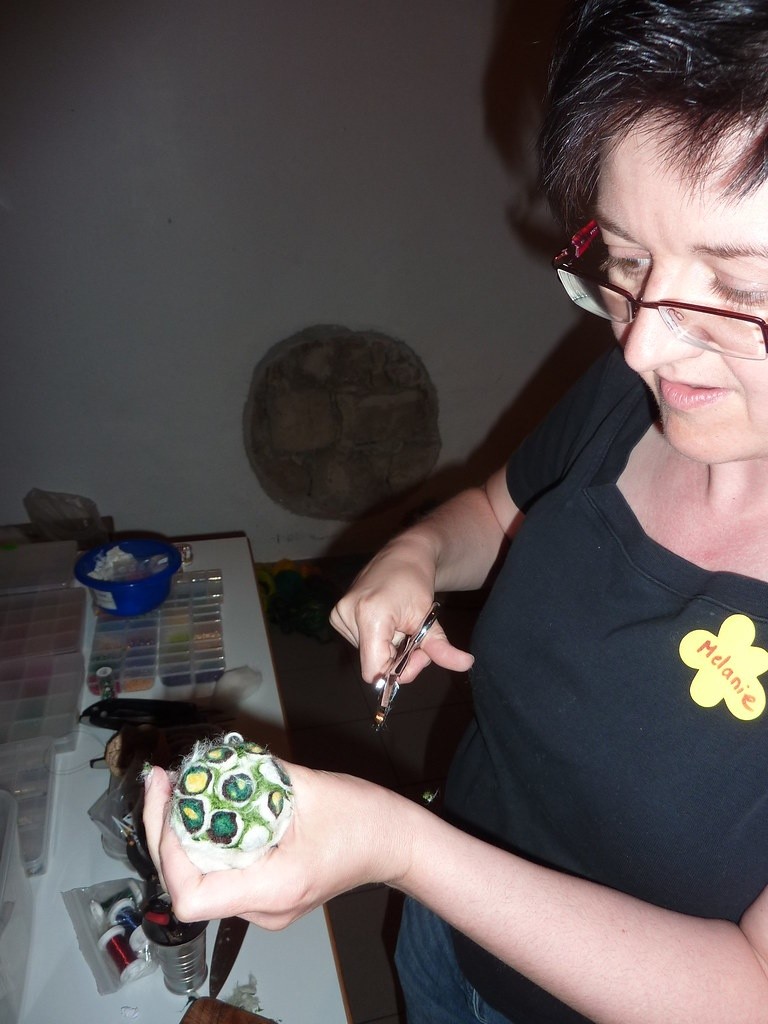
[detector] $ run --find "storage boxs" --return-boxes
[0,539,227,1024]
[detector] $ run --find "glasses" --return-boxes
[552,219,768,361]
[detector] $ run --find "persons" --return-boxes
[145,1,767,1023]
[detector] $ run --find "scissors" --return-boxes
[369,601,442,735]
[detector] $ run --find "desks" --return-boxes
[0,538,354,1024]
